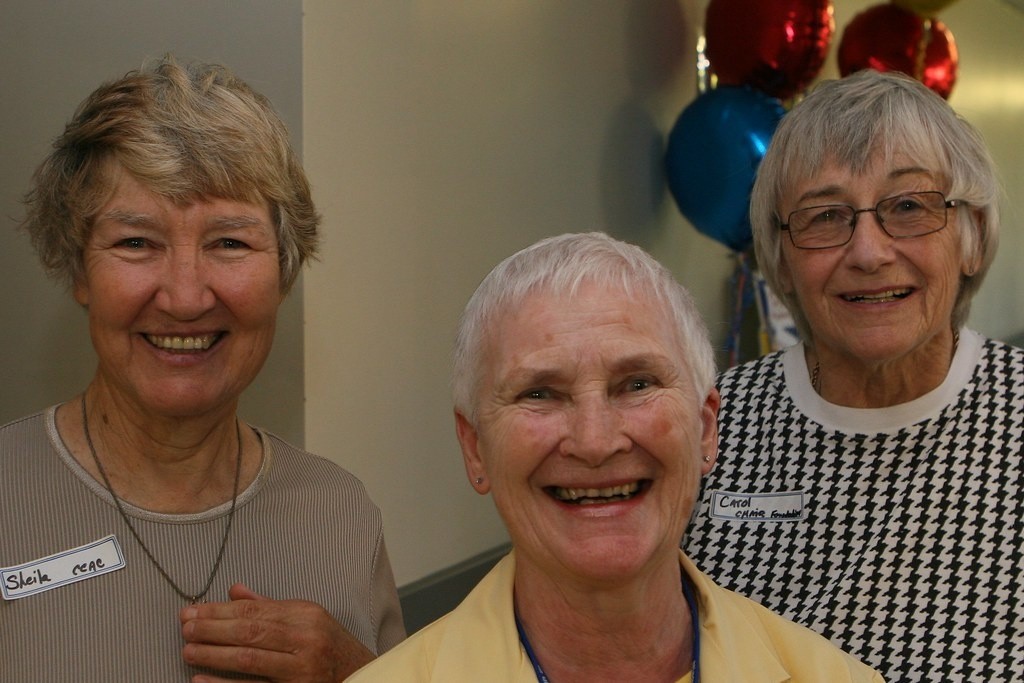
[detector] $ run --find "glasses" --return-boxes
[781,191,966,250]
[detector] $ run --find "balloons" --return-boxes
[667,0,959,263]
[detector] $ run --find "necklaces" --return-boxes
[516,581,701,683]
[811,325,959,390]
[81,390,242,605]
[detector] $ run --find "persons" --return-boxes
[676,72,1024,683]
[343,233,886,683]
[1,53,408,682]
